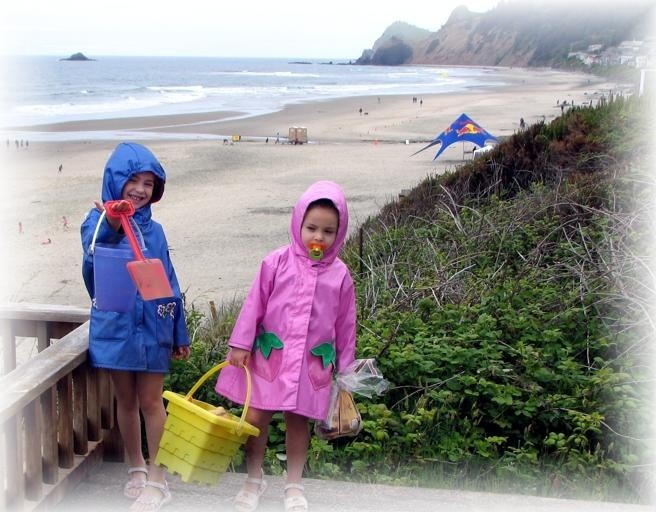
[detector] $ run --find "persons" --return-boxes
[223,138,234,145]
[58,164,63,174]
[413,97,417,103]
[266,137,268,143]
[359,108,363,115]
[421,100,423,105]
[520,118,525,128]
[275,132,279,144]
[63,216,69,230]
[80,141,191,512]
[215,180,358,512]
[18,222,23,233]
[43,239,51,244]
[557,100,574,113]
[6,139,29,149]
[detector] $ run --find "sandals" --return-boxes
[231,467,269,510]
[123,467,148,497]
[283,483,308,512]
[130,479,170,512]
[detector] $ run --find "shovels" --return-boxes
[104,199,176,303]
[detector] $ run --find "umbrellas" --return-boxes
[408,113,499,162]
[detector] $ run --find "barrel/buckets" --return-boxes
[152,362,260,488]
[88,208,147,315]
